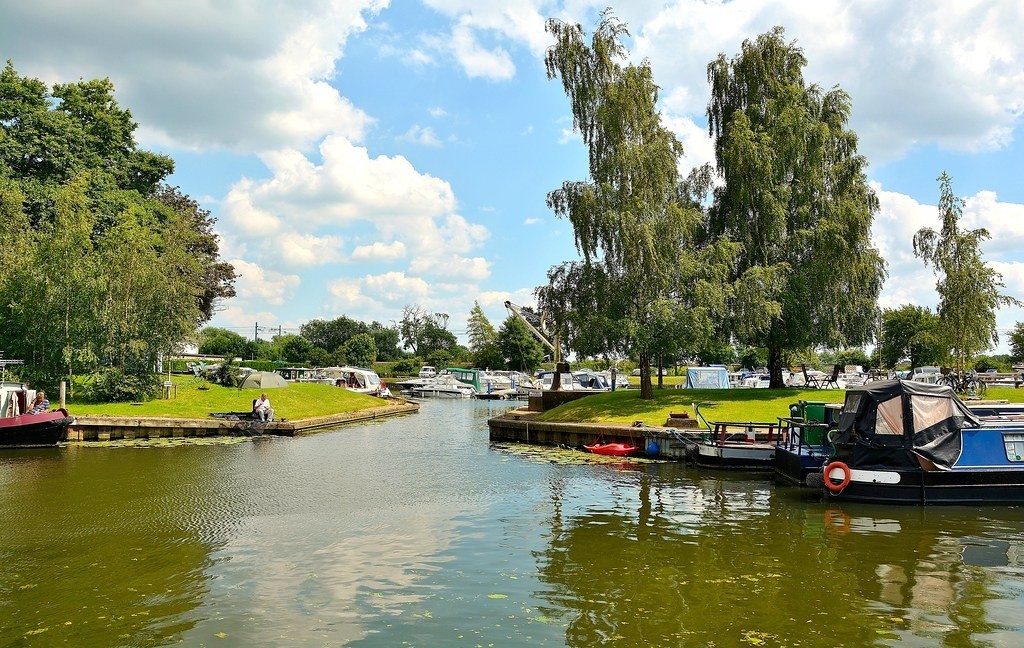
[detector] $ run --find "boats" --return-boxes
[396,365,629,401]
[682,362,868,390]
[584,442,638,456]
[769,399,844,487]
[187,359,394,397]
[806,380,1024,506]
[696,421,785,471]
[0,380,74,448]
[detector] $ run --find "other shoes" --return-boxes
[262,418,265,422]
[267,419,271,422]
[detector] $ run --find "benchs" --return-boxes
[975,373,1023,389]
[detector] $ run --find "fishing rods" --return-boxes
[264,406,281,418]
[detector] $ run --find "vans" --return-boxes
[419,367,436,378]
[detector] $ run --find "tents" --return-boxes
[237,370,289,389]
[684,367,730,389]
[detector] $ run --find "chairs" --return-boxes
[820,365,840,389]
[800,364,822,390]
[250,399,268,421]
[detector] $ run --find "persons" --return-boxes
[380,379,386,389]
[27,392,50,415]
[347,373,361,388]
[254,393,274,422]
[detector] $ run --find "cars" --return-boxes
[632,369,640,376]
[655,368,668,376]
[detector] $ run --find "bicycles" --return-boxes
[935,364,987,396]
[863,370,902,386]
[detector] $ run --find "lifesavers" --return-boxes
[824,462,850,490]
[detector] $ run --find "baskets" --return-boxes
[940,367,951,375]
[971,373,979,382]
